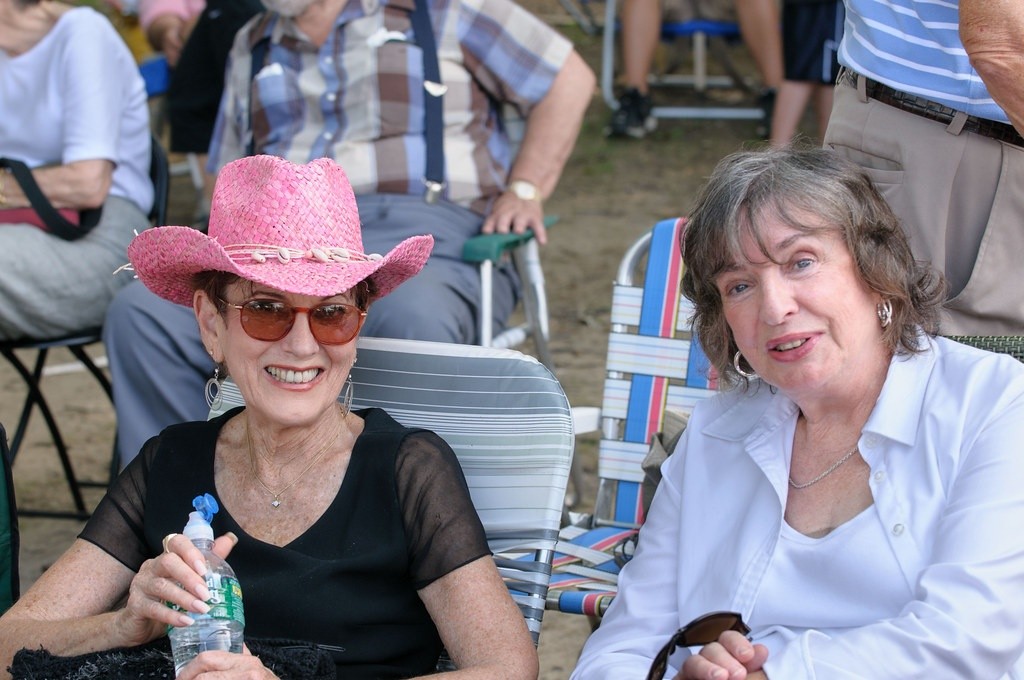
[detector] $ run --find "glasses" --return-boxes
[646,611,752,680]
[216,297,368,345]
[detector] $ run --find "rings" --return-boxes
[163,533,177,553]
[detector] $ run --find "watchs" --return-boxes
[506,180,543,205]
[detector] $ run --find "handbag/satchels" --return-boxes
[6,635,346,680]
[0,157,102,241]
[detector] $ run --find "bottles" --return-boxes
[163,493,245,676]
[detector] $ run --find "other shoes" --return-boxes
[601,88,657,139]
[756,91,776,138]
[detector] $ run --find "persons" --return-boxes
[823,0,1024,334]
[0,0,597,480]
[603,0,845,153]
[0,155,539,680]
[569,145,1024,680]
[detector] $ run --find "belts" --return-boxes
[845,68,1024,147]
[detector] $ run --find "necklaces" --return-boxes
[788,444,858,487]
[246,402,343,507]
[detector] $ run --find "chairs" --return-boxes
[209,338,575,650]
[498,211,767,636]
[599,0,773,137]
[456,101,585,510]
[0,134,174,527]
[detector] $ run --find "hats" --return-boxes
[127,155,435,307]
